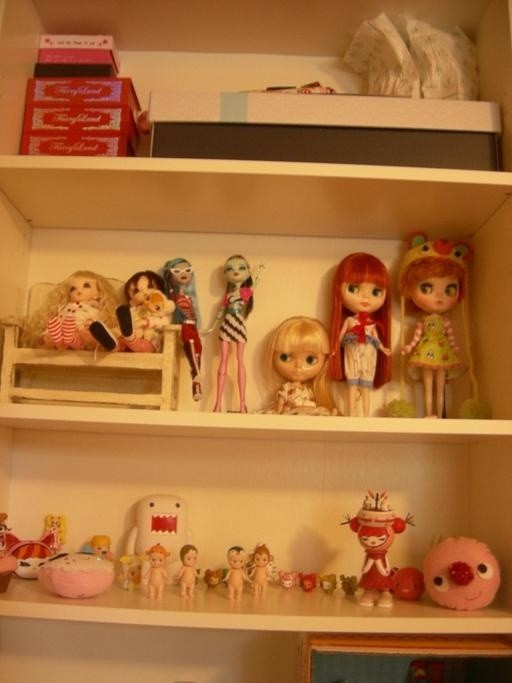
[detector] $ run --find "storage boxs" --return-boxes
[146,91,504,170]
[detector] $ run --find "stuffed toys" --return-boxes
[387,565,425,604]
[338,574,358,600]
[125,492,187,586]
[203,568,222,592]
[0,528,57,581]
[421,536,503,610]
[277,569,299,595]
[38,553,115,599]
[319,573,338,598]
[298,571,318,594]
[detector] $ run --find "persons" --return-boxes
[263,315,333,416]
[175,544,200,599]
[399,234,467,419]
[248,544,274,600]
[38,514,66,552]
[143,542,171,603]
[88,269,176,353]
[223,546,251,601]
[200,255,265,415]
[87,532,113,564]
[119,554,145,594]
[18,268,119,351]
[340,490,416,607]
[326,252,391,419]
[161,256,202,402]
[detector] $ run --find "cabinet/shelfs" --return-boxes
[1,0,511,633]
